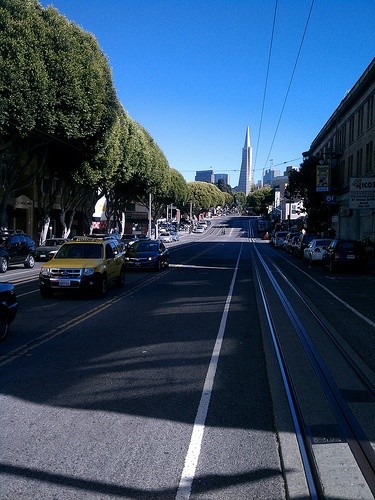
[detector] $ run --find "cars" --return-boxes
[0,281,19,340]
[122,240,170,272]
[35,238,71,262]
[321,239,368,276]
[121,234,152,250]
[151,209,231,243]
[270,231,322,259]
[304,239,333,264]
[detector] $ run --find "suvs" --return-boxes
[39,235,126,300]
[0,229,37,273]
